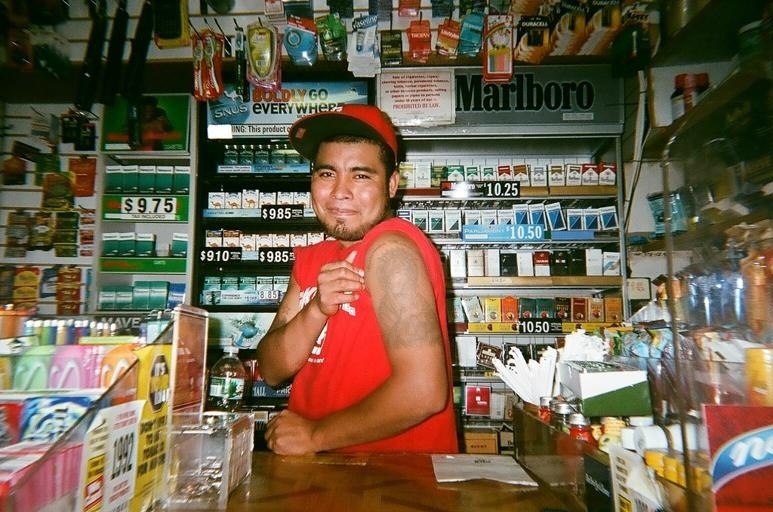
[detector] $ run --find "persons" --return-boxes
[253,103,459,457]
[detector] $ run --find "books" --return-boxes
[429,453,539,488]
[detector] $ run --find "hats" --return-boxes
[289,104,398,162]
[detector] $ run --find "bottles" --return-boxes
[209,346,246,413]
[538,397,682,498]
